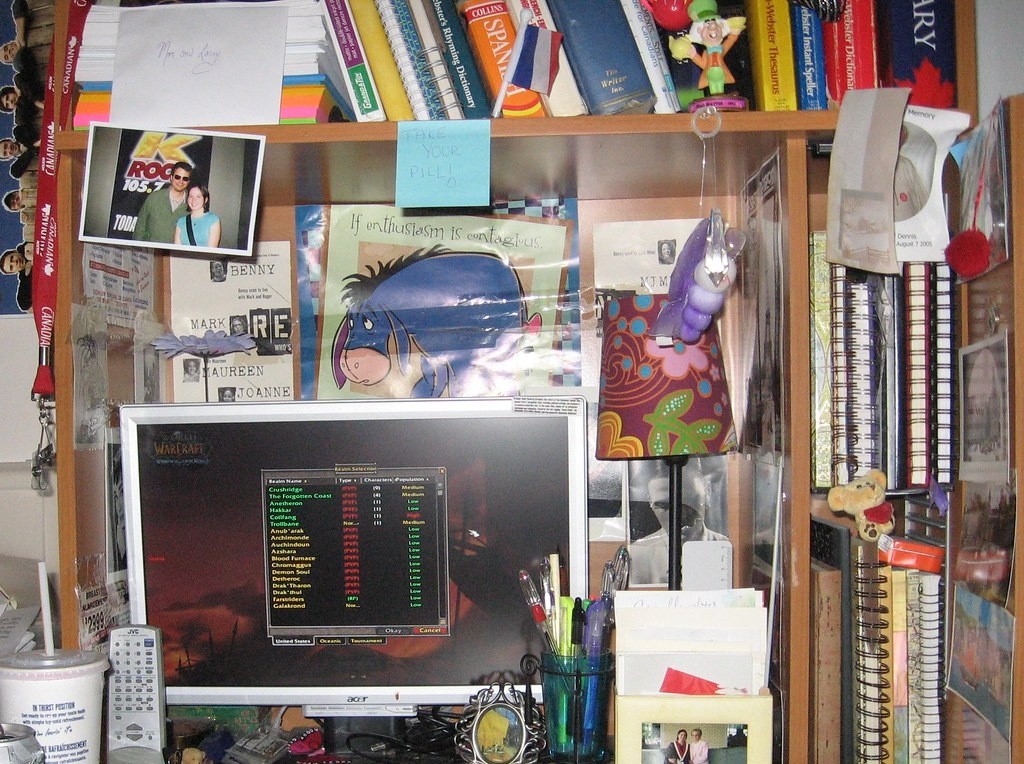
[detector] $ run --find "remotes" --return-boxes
[105,625,165,764]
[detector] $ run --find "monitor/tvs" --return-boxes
[118,393,589,755]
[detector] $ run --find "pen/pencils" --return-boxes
[572,595,587,763]
[581,544,632,755]
[541,543,575,755]
[518,569,572,693]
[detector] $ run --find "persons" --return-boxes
[0,241,36,312]
[2,190,29,223]
[0,72,45,127]
[212,261,226,281]
[231,316,246,337]
[659,240,676,264]
[184,358,199,382]
[687,728,710,764]
[664,728,692,764]
[219,388,235,403]
[173,183,222,249]
[0,0,37,75]
[628,459,729,588]
[1,125,42,181]
[131,161,193,245]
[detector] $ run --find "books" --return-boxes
[316,0,960,128]
[804,511,947,764]
[805,229,962,496]
